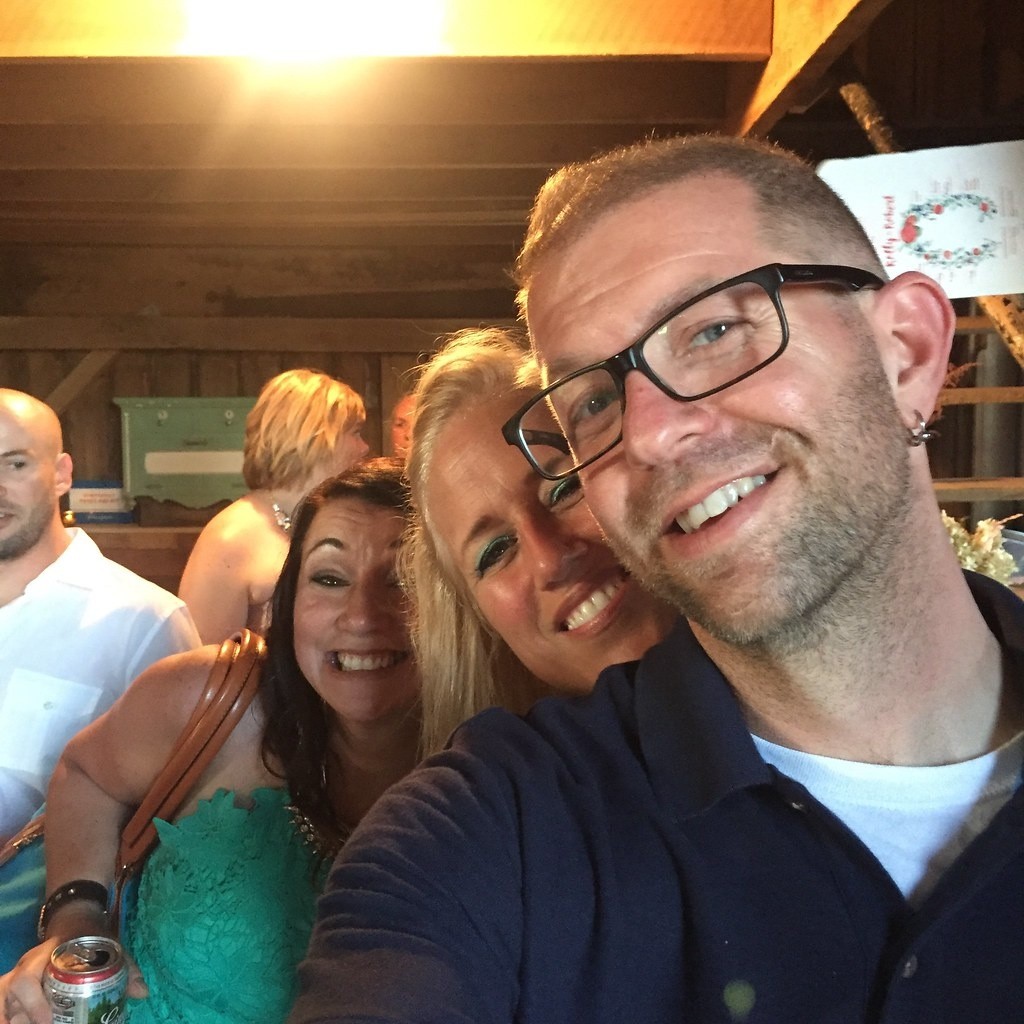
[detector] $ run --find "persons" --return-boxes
[0,387,203,856]
[395,324,680,745]
[389,392,419,460]
[289,135,1024,1024]
[178,369,369,648]
[0,456,425,1024]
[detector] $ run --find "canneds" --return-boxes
[41,936,130,1024]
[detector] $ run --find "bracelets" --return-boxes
[37,880,108,939]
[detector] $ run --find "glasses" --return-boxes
[499,262,888,481]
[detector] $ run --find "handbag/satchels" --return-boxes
[0,627,269,976]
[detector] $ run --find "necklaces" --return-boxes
[281,723,345,861]
[267,493,292,535]
